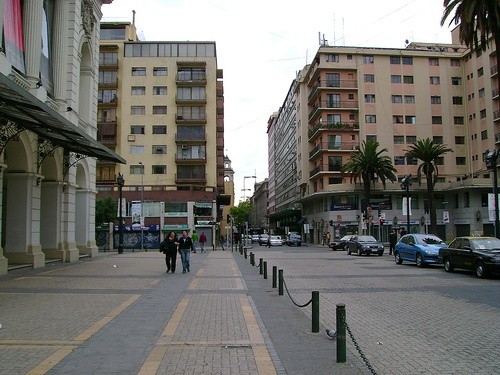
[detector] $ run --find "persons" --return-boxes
[199,231,207,254]
[266,236,272,248]
[191,230,198,253]
[388,230,396,255]
[326,232,331,244]
[163,231,180,273]
[322,232,328,246]
[400,227,406,237]
[179,230,193,273]
[220,235,226,251]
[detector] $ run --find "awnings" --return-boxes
[0,72,126,183]
[298,218,306,224]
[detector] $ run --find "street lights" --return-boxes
[117,171,125,255]
[401,173,413,234]
[482,148,500,239]
[138,161,144,251]
[243,176,257,246]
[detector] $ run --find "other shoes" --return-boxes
[172,271,175,274]
[166,269,170,272]
[183,270,186,274]
[187,269,190,272]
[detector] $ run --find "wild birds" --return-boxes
[113,264,118,268]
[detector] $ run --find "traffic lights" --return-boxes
[378,208,382,217]
[227,214,231,223]
[193,205,197,214]
[329,220,333,226]
[425,199,431,214]
[381,219,385,225]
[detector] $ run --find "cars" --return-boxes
[394,234,448,267]
[347,235,384,256]
[242,233,283,246]
[329,235,356,251]
[438,236,500,279]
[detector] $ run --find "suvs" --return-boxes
[286,232,302,247]
